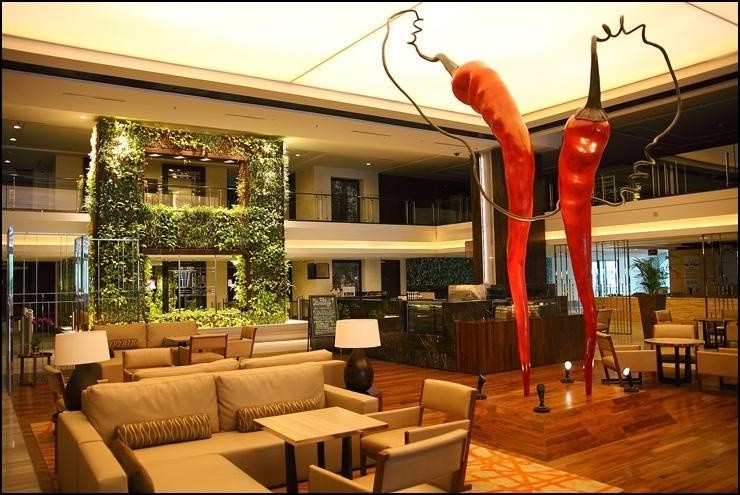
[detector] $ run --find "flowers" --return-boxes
[32,317,56,348]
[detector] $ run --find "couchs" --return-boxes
[44,319,389,492]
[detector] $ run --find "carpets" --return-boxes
[29,421,58,493]
[269,437,626,494]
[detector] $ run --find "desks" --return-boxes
[18,352,53,388]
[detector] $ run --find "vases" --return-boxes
[32,347,40,355]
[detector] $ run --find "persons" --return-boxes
[330,280,341,297]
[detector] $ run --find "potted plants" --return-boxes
[631,256,669,338]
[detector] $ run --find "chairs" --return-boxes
[358,378,477,494]
[597,306,740,393]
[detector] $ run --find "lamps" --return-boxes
[623,367,639,393]
[533,384,551,413]
[560,361,575,383]
[475,374,489,401]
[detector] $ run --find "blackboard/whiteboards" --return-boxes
[312,297,337,335]
